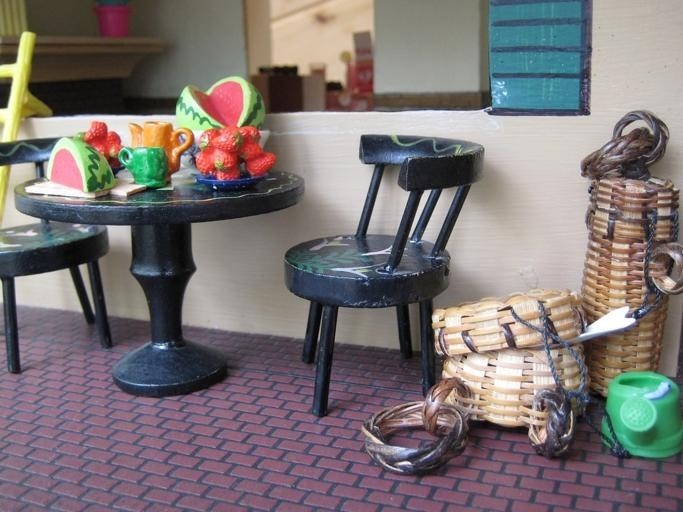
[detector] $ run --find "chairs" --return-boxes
[282,134,486,417]
[0,140,114,375]
